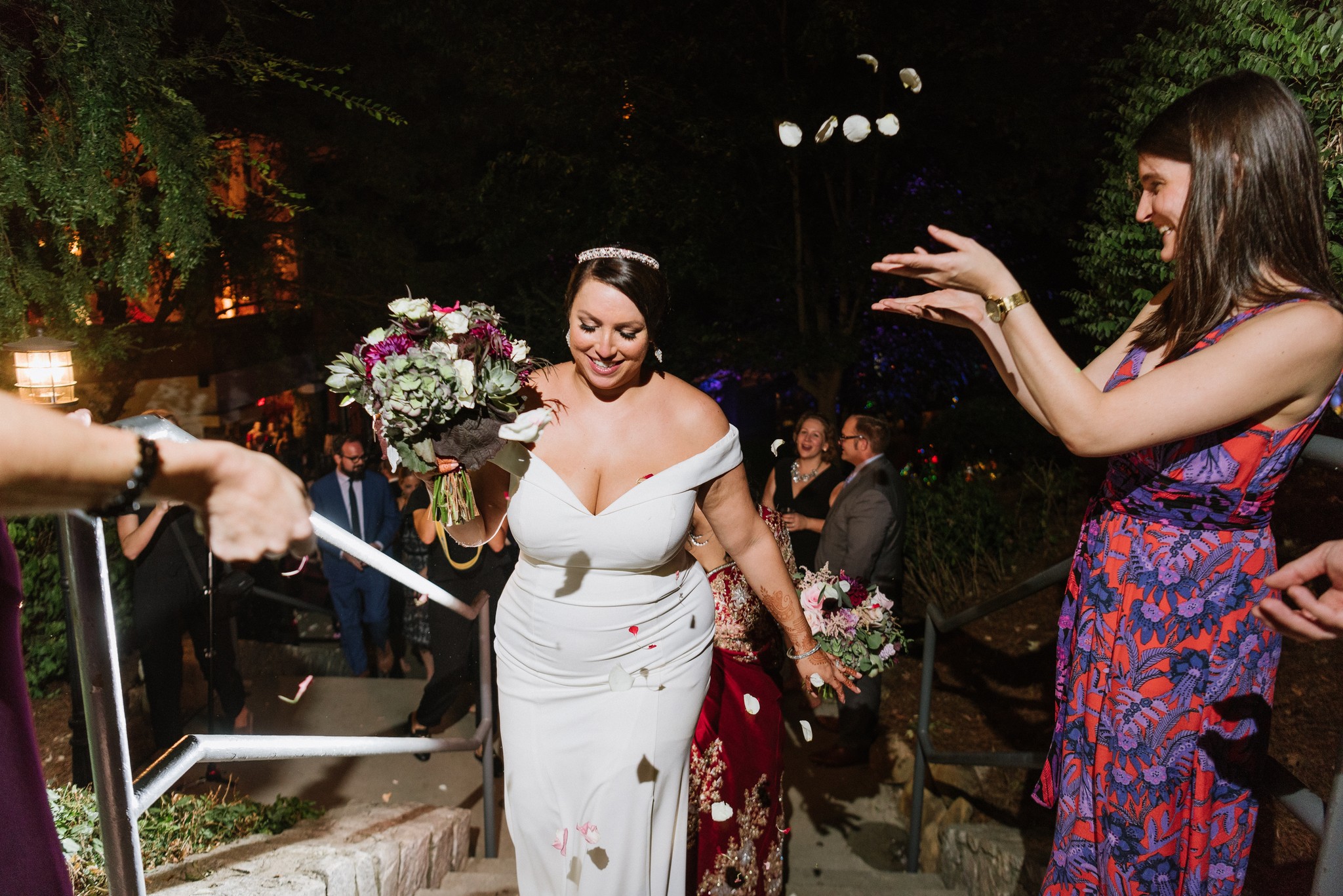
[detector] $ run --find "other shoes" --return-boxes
[410,713,431,765]
[473,745,504,776]
[814,746,867,770]
[228,710,252,735]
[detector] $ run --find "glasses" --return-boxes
[839,434,868,443]
[338,453,368,463]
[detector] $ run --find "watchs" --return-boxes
[986,290,1030,323]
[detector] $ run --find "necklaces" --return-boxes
[790,460,821,483]
[687,530,712,547]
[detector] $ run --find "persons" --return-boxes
[812,414,903,767]
[760,411,854,577]
[306,432,519,775]
[0,392,315,896]
[687,494,803,896]
[116,407,250,754]
[1252,541,1343,896]
[398,249,862,896]
[871,69,1343,896]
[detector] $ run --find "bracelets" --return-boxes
[86,439,162,520]
[787,638,821,659]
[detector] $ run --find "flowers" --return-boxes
[319,282,572,531]
[788,558,915,702]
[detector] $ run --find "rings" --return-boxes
[836,659,839,667]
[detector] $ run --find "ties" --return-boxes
[348,480,361,540]
